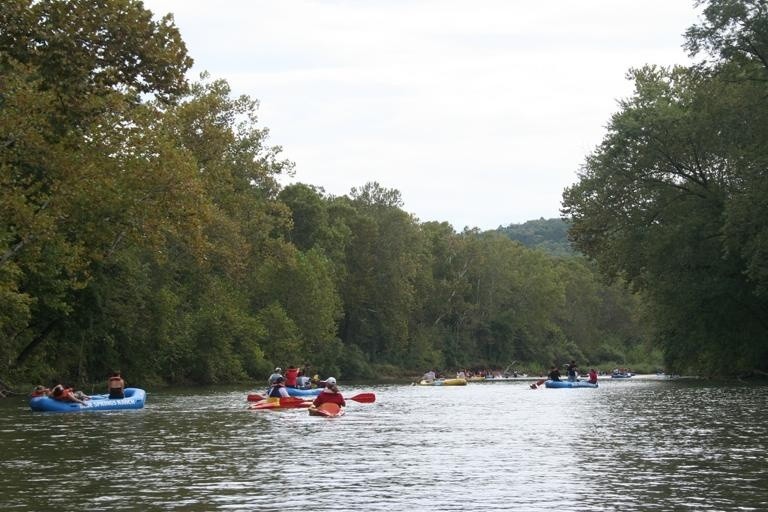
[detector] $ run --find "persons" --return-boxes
[297,371,312,389]
[51,383,91,407]
[268,376,290,398]
[268,366,284,384]
[421,366,633,384]
[285,365,297,387]
[36,385,51,394]
[312,377,346,408]
[108,370,125,398]
[566,360,577,382]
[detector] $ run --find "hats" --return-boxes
[326,377,336,384]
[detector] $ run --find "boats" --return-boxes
[243,378,352,418]
[410,367,638,389]
[29,386,145,411]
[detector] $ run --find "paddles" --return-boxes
[249,394,265,402]
[279,393,376,409]
[537,378,549,386]
